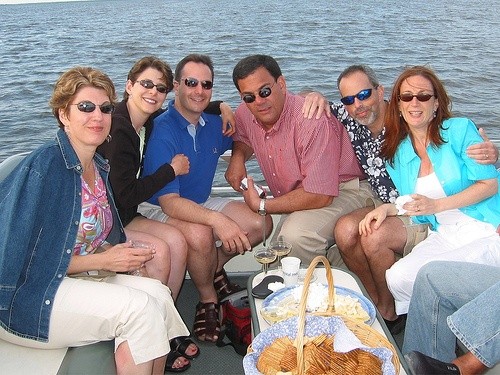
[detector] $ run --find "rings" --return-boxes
[486,155,489,159]
[417,206,420,212]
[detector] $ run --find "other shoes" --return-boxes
[382,315,401,330]
[404,350,460,375]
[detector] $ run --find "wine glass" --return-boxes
[254,248,278,280]
[130,239,156,278]
[402,193,419,227]
[267,241,294,273]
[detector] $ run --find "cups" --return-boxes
[279,256,301,275]
[297,264,318,283]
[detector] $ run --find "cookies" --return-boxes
[256,333,383,375]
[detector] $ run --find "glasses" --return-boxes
[178,77,215,90]
[133,79,169,95]
[398,92,435,102]
[341,85,380,105]
[240,81,275,103]
[68,100,115,114]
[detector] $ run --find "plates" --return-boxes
[260,282,376,327]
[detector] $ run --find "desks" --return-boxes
[247,265,411,375]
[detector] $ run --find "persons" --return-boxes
[225,54,374,266]
[142,55,274,344]
[402,224,500,375]
[358,65,500,315]
[299,64,500,334]
[96,57,237,372]
[0,67,191,375]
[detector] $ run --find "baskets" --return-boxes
[242,256,400,375]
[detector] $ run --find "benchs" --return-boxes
[0,152,281,375]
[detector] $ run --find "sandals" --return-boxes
[170,336,200,359]
[192,302,221,345]
[165,351,191,372]
[214,269,248,303]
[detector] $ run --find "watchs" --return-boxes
[257,199,267,216]
